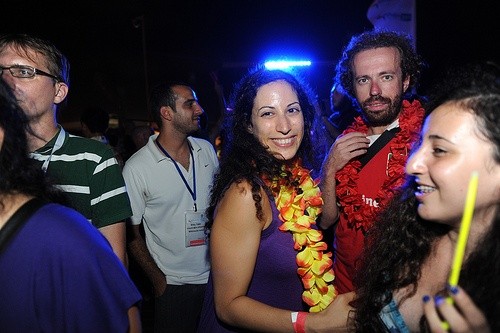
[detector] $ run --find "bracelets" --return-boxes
[291,310,309,333]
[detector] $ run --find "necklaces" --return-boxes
[330,98,426,234]
[249,156,339,315]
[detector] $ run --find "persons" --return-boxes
[122,78,222,333]
[197,70,369,333]
[55,64,360,167]
[346,81,500,333]
[1,33,134,269]
[315,31,431,311]
[0,73,143,333]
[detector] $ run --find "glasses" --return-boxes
[1,64,60,81]
